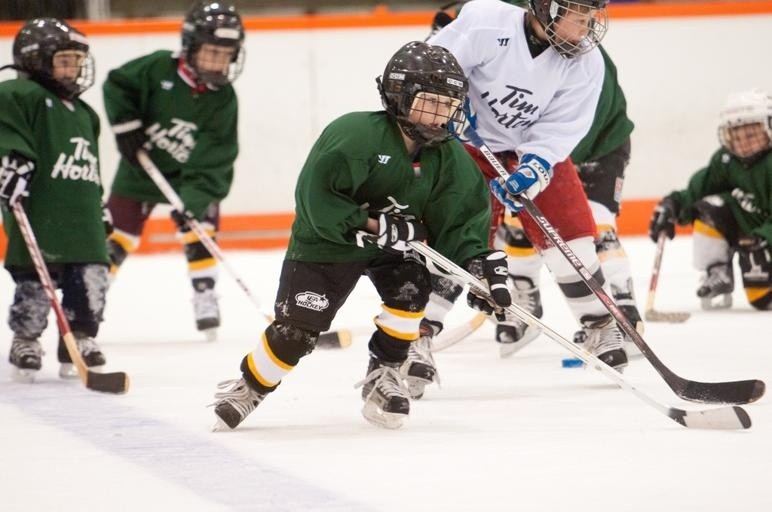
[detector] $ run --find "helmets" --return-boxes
[377,41,472,147]
[178,2,245,89]
[717,88,772,158]
[525,0,608,60]
[12,16,96,97]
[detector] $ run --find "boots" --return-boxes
[559,315,627,368]
[494,277,544,344]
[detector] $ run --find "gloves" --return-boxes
[376,211,430,253]
[113,120,148,167]
[447,95,479,145]
[171,204,196,232]
[489,157,552,214]
[100,200,114,239]
[649,192,678,242]
[466,249,512,316]
[0,154,36,204]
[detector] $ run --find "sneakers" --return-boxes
[57,333,105,369]
[618,301,645,341]
[8,332,44,370]
[362,355,411,414]
[216,370,267,429]
[402,337,437,384]
[194,290,222,329]
[697,262,735,296]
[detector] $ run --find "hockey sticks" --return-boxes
[13,203,128,393]
[432,310,484,349]
[463,124,765,403]
[644,233,690,323]
[138,150,352,350]
[411,240,752,430]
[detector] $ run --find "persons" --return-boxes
[649,92,772,309]
[398,0,629,384]
[102,1,247,330]
[425,0,643,345]
[0,18,109,370]
[205,40,511,428]
